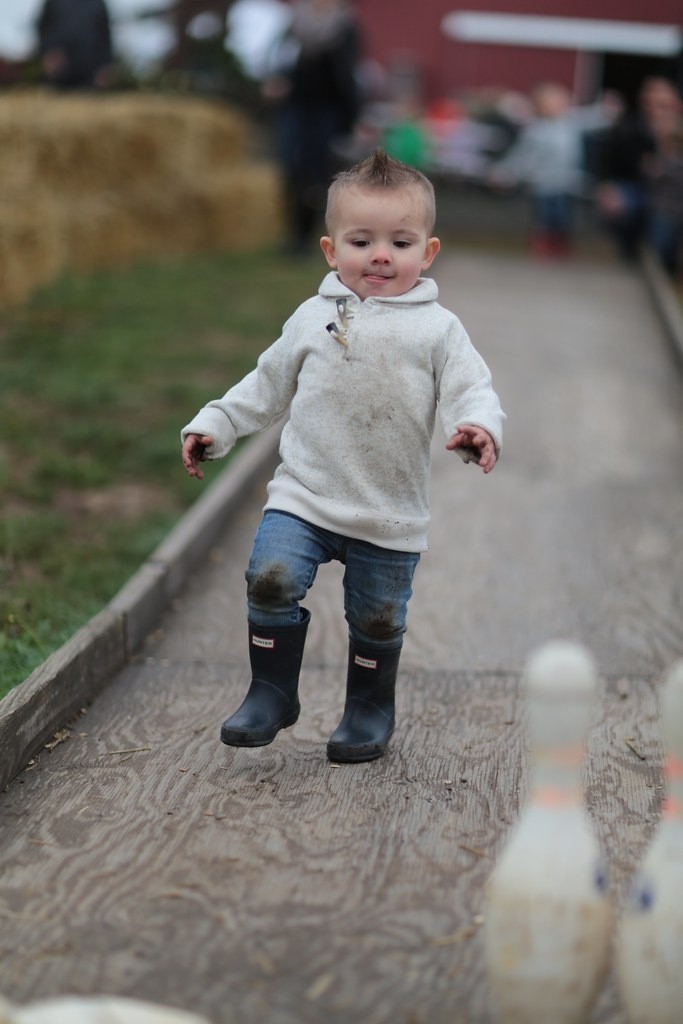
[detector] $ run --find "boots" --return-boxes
[325,635,404,764]
[219,606,312,749]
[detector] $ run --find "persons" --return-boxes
[27,0,683,278]
[178,149,506,763]
